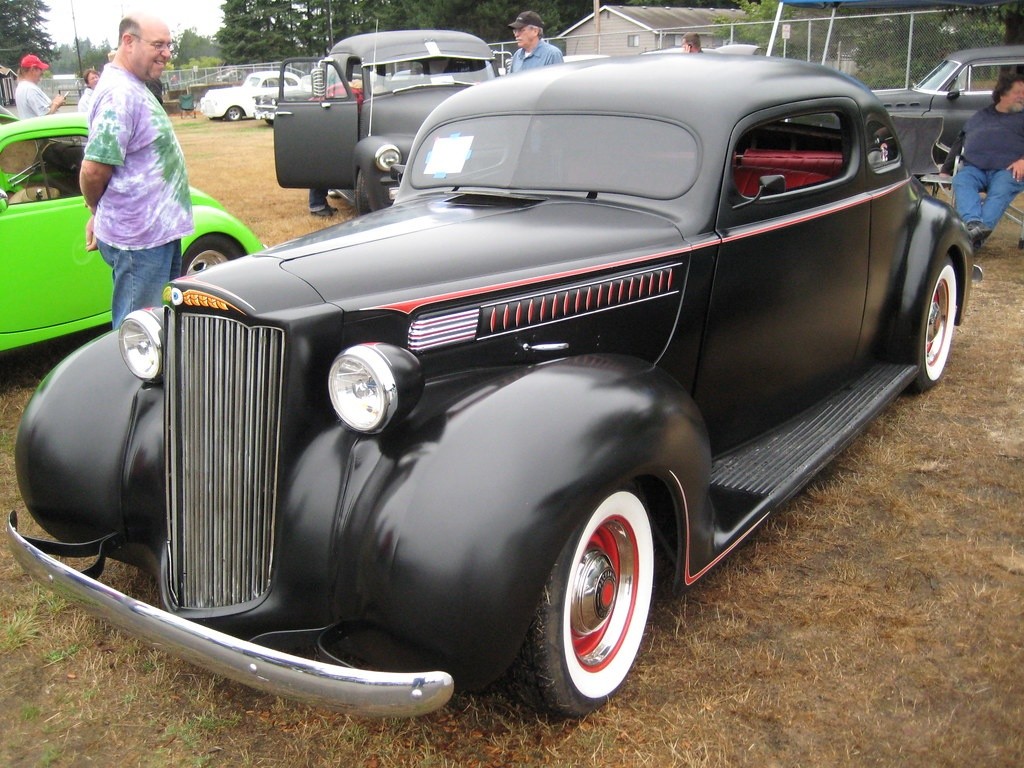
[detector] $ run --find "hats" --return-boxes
[507,10,544,30]
[21,54,50,69]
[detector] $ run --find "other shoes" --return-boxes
[969,223,992,245]
[311,208,333,218]
[325,204,338,215]
[968,221,983,250]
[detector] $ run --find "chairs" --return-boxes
[732,147,847,202]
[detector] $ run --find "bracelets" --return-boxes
[53,102,60,106]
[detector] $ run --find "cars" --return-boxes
[0,113,266,366]
[272,27,506,218]
[8,58,981,723]
[865,44,1024,164]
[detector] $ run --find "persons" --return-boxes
[79,68,101,111]
[510,10,564,74]
[940,73,1024,254]
[681,32,703,53]
[171,75,178,90]
[108,47,163,105]
[302,77,385,217]
[15,55,66,121]
[78,11,191,327]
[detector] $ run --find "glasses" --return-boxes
[129,34,176,51]
[512,28,525,33]
